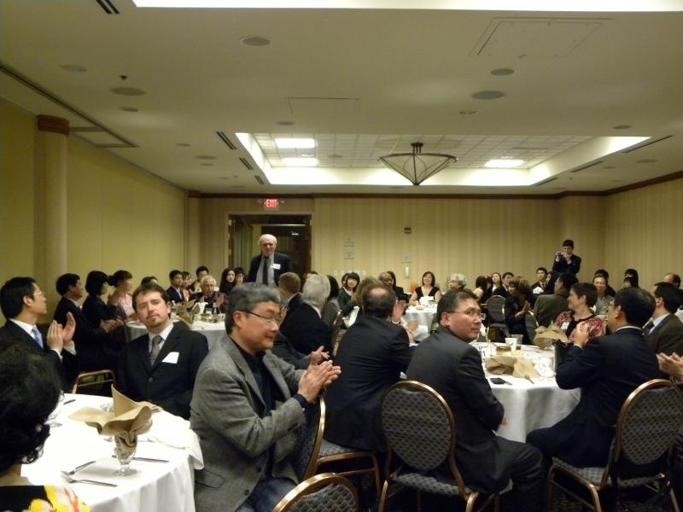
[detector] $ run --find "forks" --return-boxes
[58,470,117,488]
[60,460,96,475]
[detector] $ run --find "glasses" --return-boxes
[236,308,282,324]
[448,310,487,321]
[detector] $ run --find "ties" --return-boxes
[148,335,164,369]
[262,256,269,287]
[643,320,655,334]
[31,327,43,348]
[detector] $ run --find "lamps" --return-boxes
[380,143,457,186]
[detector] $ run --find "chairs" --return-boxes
[315,439,384,502]
[379,380,512,512]
[73,370,116,394]
[546,379,683,512]
[272,473,360,512]
[293,394,326,481]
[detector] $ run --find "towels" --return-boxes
[68,383,161,462]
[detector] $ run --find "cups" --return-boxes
[510,334,523,350]
[46,391,65,428]
[112,435,138,477]
[505,337,517,351]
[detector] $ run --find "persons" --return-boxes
[0,277,79,392]
[544,271,559,294]
[142,276,158,284]
[220,268,235,296]
[554,283,604,342]
[662,273,680,289]
[625,269,639,288]
[166,270,191,304]
[473,274,493,304]
[409,271,442,306]
[81,270,121,323]
[248,234,293,287]
[337,272,360,316]
[533,273,578,328]
[108,270,135,316]
[0,341,92,512]
[595,269,616,297]
[531,267,548,289]
[378,271,401,301]
[526,287,660,474]
[54,274,124,339]
[190,282,342,512]
[200,275,216,296]
[504,276,537,345]
[115,282,208,418]
[278,272,303,309]
[494,272,514,298]
[196,266,209,281]
[491,272,508,298]
[592,275,615,315]
[656,352,683,378]
[447,273,467,290]
[279,274,337,365]
[642,282,683,358]
[406,288,544,512]
[234,268,245,285]
[323,283,413,453]
[553,240,581,276]
[342,273,349,284]
[624,277,634,288]
